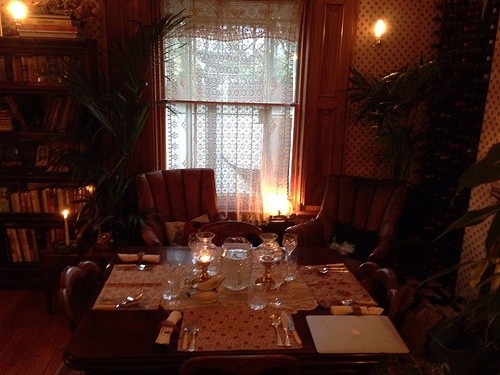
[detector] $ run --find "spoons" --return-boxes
[272,315,284,346]
[182,324,189,349]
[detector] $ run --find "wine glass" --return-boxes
[271,263,285,304]
[282,233,297,262]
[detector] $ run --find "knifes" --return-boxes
[288,316,301,344]
[281,311,291,346]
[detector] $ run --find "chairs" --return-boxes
[179,355,301,375]
[58,260,103,335]
[135,169,236,247]
[285,174,408,261]
[193,221,266,247]
[354,262,401,318]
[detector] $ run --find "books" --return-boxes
[0,186,96,263]
[0,54,83,83]
[15,13,79,40]
[0,87,79,131]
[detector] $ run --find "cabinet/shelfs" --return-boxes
[0,38,98,288]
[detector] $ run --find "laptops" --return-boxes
[306,315,409,354]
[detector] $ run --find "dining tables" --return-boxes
[61,244,409,374]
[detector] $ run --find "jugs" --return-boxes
[221,237,251,290]
[254,233,286,290]
[190,232,221,276]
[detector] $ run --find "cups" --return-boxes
[163,263,181,299]
[283,255,296,281]
[249,279,267,310]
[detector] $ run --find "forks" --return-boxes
[188,324,199,351]
